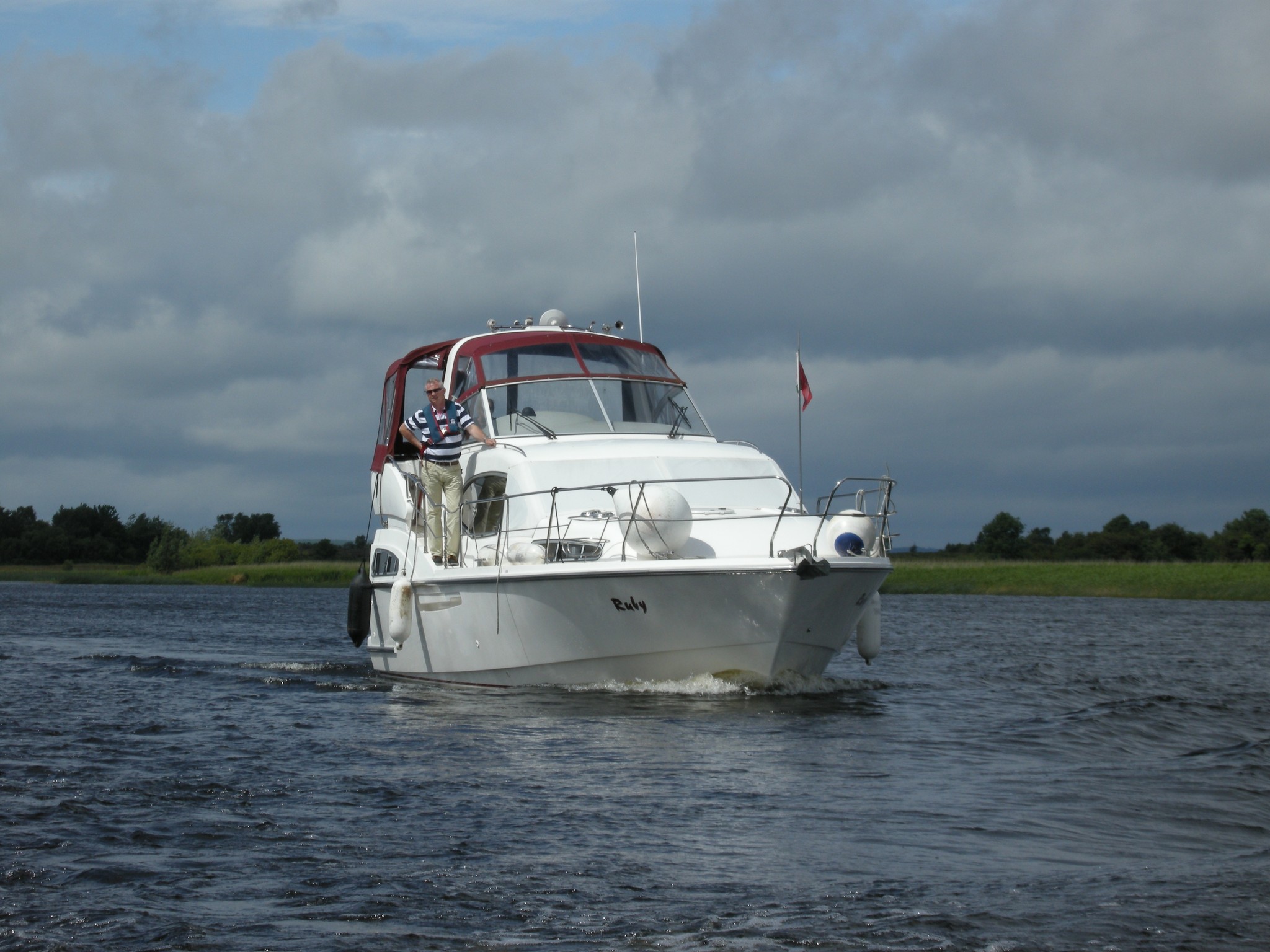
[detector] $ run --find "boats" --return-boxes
[344,230,901,694]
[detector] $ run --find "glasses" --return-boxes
[424,388,443,395]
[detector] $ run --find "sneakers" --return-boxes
[432,556,443,565]
[448,555,459,566]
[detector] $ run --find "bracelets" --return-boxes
[483,437,490,442]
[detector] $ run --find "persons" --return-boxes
[472,398,495,429]
[399,378,496,566]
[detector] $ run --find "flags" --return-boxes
[797,361,812,412]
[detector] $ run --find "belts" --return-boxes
[423,457,459,466]
